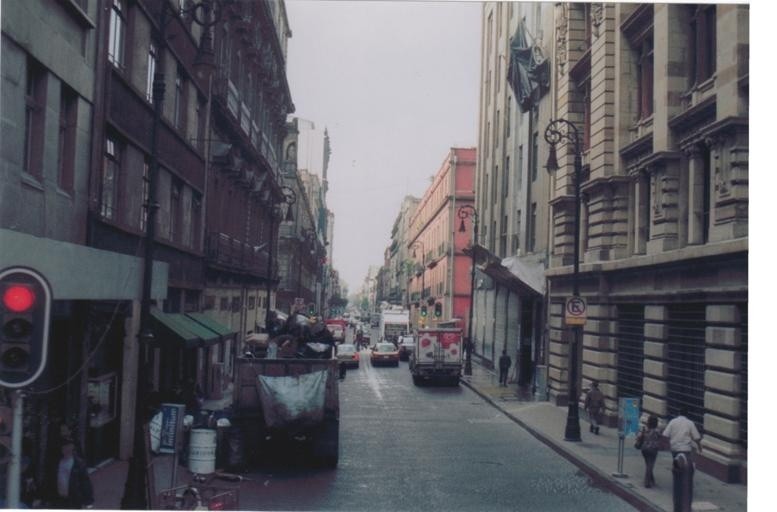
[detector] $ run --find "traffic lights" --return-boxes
[0,266,49,389]
[435,303,442,316]
[308,304,316,315]
[421,306,427,316]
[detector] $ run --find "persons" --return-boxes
[45,408,95,508]
[499,350,511,387]
[264,310,340,359]
[584,379,605,434]
[384,330,405,350]
[353,330,367,353]
[634,415,662,488]
[661,407,702,512]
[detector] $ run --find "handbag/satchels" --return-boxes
[635,437,643,449]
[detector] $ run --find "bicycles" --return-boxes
[159,472,249,511]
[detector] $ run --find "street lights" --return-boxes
[264,185,297,331]
[458,204,479,377]
[294,227,317,300]
[406,240,425,302]
[121,0,221,510]
[544,116,583,444]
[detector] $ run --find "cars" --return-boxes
[326,309,419,369]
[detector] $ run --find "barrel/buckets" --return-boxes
[187,428,217,474]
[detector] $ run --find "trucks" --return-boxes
[228,346,339,468]
[409,328,463,387]
